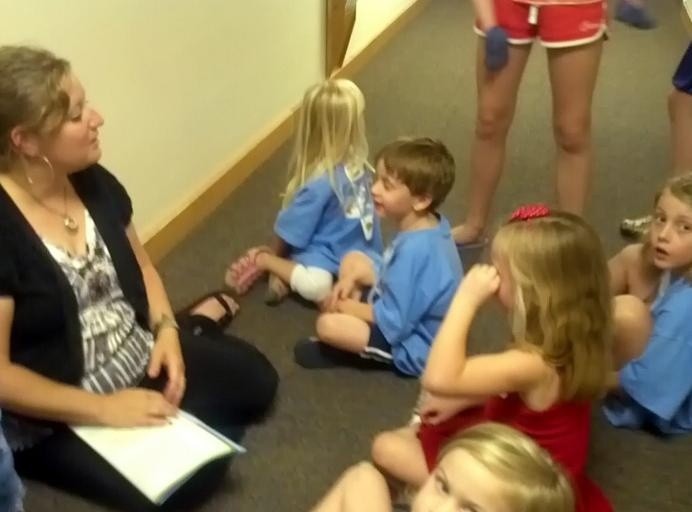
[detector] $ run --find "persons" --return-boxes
[372,206,620,512]
[293,137,465,377]
[621,42,692,240]
[0,46,277,512]
[225,80,383,305]
[446,0,609,248]
[307,422,574,512]
[607,173,691,435]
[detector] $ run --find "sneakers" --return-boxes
[620,216,654,240]
[484,25,508,70]
[294,335,363,371]
[613,2,654,31]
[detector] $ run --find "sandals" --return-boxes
[266,273,291,305]
[226,242,272,299]
[175,289,241,332]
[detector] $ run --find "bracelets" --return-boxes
[152,315,182,340]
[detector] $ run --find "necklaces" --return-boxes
[12,177,79,231]
